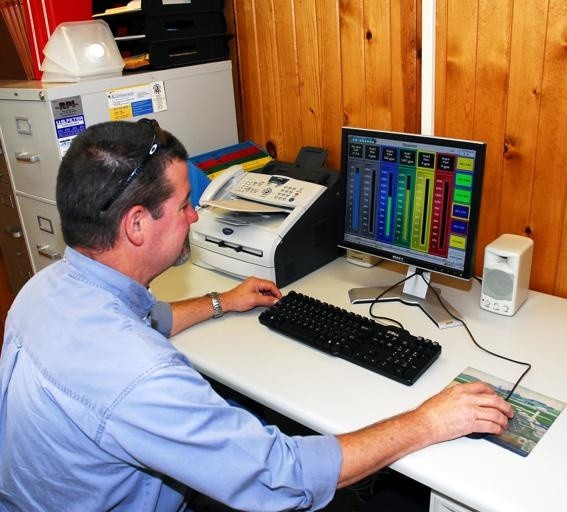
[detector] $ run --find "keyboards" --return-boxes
[258,290,441,386]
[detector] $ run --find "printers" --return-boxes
[187,145,347,291]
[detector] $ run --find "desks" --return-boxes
[148,252,567,511]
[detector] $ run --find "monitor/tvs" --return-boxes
[336,126,487,329]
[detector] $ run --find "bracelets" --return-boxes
[206,290,224,320]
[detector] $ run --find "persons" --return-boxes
[1,117,516,511]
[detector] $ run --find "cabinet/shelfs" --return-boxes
[0,139,33,298]
[0,59,239,276]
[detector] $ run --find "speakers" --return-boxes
[344,248,386,268]
[482,232,535,316]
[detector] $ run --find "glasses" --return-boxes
[101,117,169,212]
